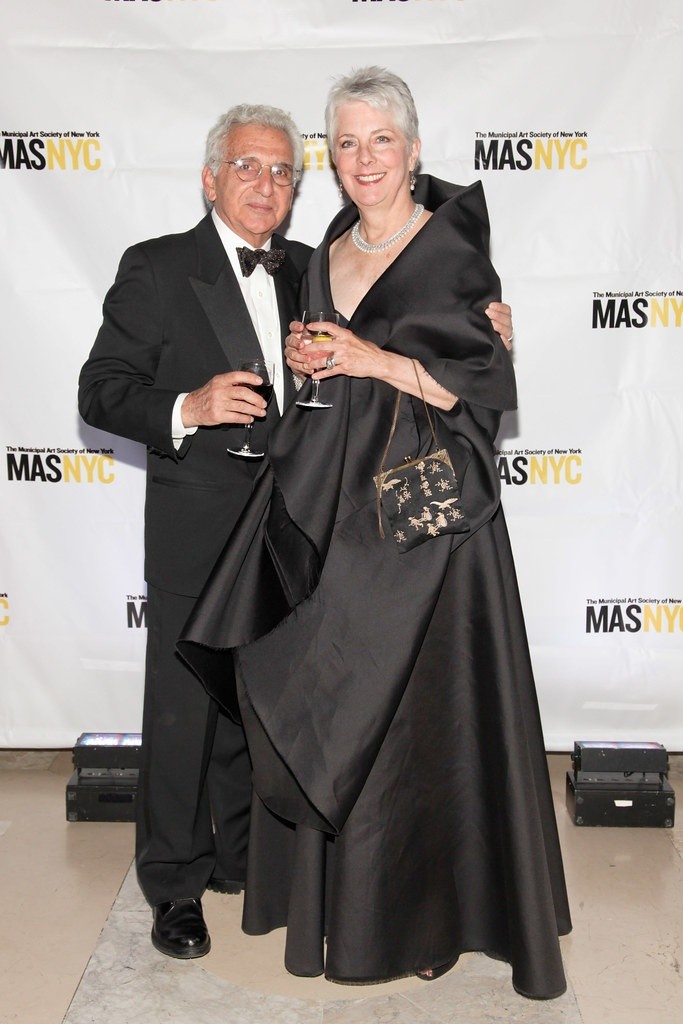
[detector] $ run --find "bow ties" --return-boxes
[236,245,286,278]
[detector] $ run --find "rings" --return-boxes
[508,333,514,341]
[327,356,333,369]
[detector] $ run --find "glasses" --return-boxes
[213,158,302,187]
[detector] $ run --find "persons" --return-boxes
[175,67,574,1001]
[78,104,514,958]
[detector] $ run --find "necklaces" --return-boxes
[351,204,424,252]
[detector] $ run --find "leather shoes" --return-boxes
[150,898,211,959]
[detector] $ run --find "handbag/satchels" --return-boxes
[372,359,471,554]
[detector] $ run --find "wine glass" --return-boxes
[226,359,275,458]
[296,310,340,408]
